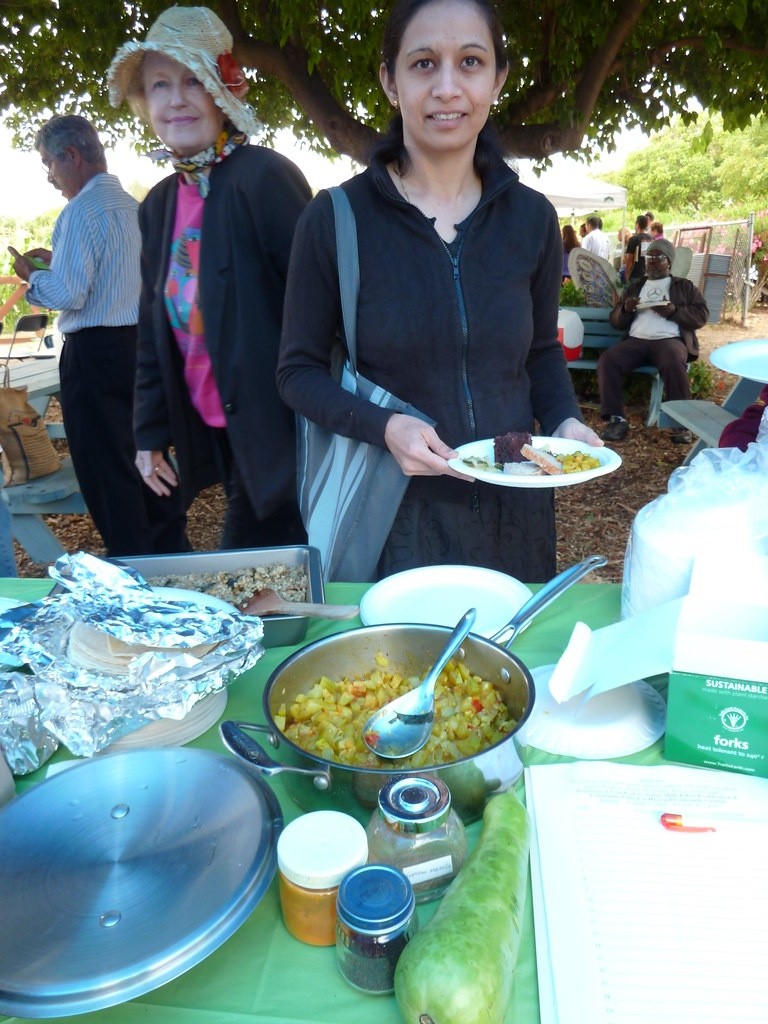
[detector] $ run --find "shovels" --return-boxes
[236,588,359,620]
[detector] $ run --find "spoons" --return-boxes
[363,608,477,758]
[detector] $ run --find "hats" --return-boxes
[646,239,675,265]
[107,7,265,136]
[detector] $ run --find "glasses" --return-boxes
[40,144,73,174]
[646,254,668,261]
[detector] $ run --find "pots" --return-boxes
[220,553,607,825]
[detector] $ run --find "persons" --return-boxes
[614,210,664,287]
[103,6,315,552]
[7,112,196,556]
[578,216,609,301]
[279,0,603,585]
[596,238,709,446]
[562,224,581,283]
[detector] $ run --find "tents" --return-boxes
[512,157,629,269]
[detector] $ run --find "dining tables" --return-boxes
[682,340,768,465]
[0,578,670,1024]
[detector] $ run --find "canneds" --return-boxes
[277,809,369,947]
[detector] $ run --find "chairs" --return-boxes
[0,314,48,368]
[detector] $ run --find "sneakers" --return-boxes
[601,416,629,441]
[668,427,693,443]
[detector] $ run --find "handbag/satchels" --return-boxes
[297,186,436,583]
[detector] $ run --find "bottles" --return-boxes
[338,865,418,996]
[275,809,369,947]
[367,772,470,904]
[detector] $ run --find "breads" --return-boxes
[493,431,563,476]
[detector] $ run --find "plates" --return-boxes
[621,493,768,618]
[447,436,623,488]
[636,300,671,308]
[520,660,668,758]
[362,564,534,644]
[53,586,231,761]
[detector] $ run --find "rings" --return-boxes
[153,466,159,473]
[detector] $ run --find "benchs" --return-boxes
[5,377,90,563]
[560,306,662,426]
[662,399,740,447]
